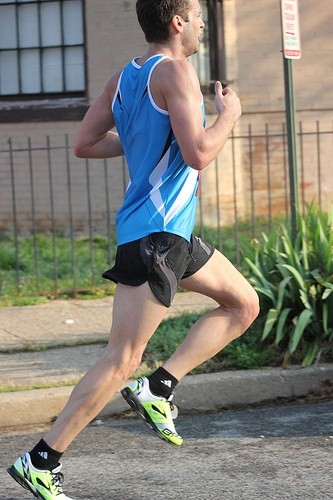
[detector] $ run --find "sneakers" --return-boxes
[120,376,184,446]
[7,451,74,500]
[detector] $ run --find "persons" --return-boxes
[7,0,260,500]
